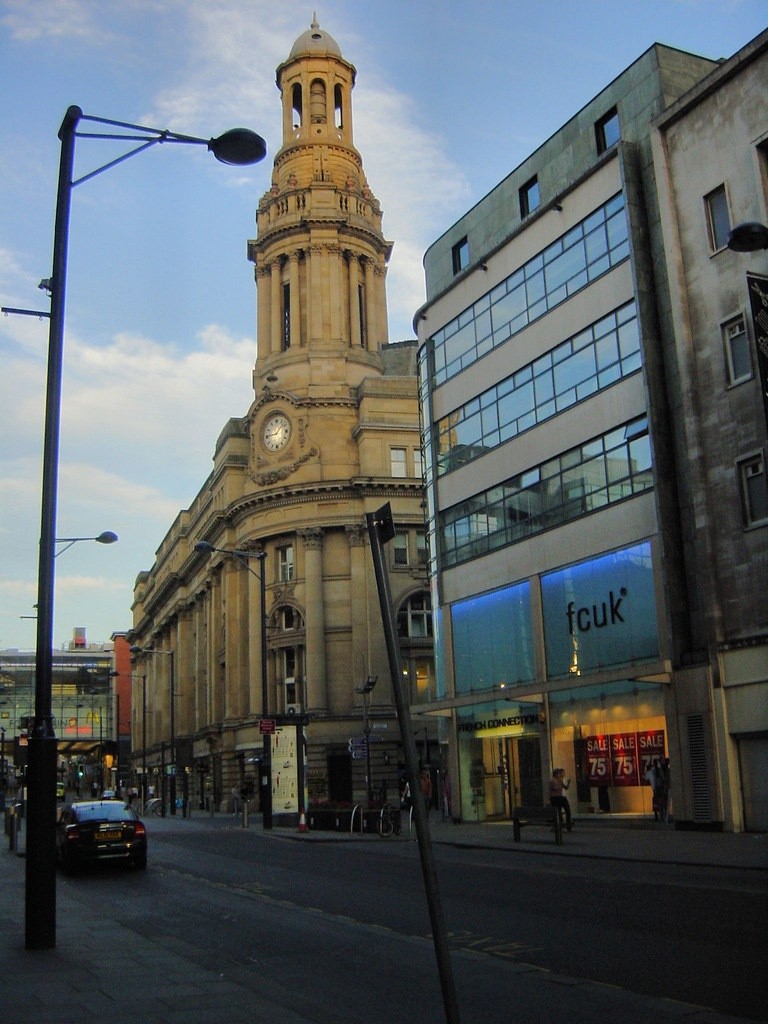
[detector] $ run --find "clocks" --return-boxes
[264,416,291,452]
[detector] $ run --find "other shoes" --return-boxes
[239,812,242,814]
[233,813,235,816]
[551,827,556,832]
[567,827,571,832]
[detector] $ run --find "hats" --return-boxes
[422,770,427,774]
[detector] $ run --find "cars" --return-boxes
[101,790,125,801]
[58,800,148,878]
[56,782,65,801]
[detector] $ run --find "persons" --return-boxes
[231,784,250,815]
[644,758,672,822]
[422,772,431,820]
[93,780,98,798]
[401,782,415,821]
[548,768,575,832]
[597,765,610,812]
[127,784,156,802]
[469,760,485,788]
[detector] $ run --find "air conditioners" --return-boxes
[285,703,301,715]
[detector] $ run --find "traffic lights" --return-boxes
[78,771,84,778]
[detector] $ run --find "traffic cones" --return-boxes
[296,809,310,834]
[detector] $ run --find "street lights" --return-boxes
[61,761,72,783]
[108,671,148,818]
[129,645,176,815]
[23,106,270,948]
[194,543,278,828]
[37,530,120,557]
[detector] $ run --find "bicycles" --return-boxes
[136,798,167,818]
[377,803,401,838]
